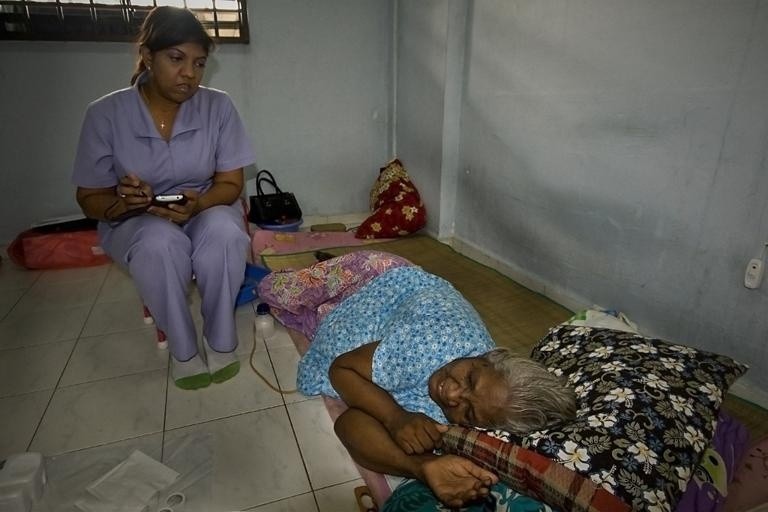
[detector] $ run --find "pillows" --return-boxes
[474,325,750,512]
[354,158,427,239]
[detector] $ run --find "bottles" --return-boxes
[255,302,274,338]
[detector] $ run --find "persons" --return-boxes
[257,250,576,509]
[71,7,256,389]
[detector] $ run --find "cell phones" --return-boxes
[152,193,187,208]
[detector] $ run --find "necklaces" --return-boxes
[140,84,178,129]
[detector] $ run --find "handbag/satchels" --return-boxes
[246,170,303,224]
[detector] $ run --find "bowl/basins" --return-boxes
[259,220,302,233]
